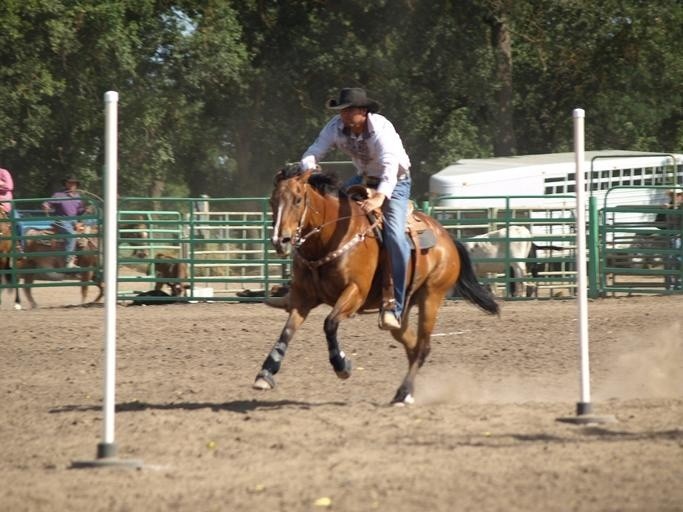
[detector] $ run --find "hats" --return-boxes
[60,174,81,187]
[665,185,683,197]
[325,87,380,113]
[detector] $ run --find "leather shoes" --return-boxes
[381,310,401,328]
[66,262,81,269]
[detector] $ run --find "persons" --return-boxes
[0,167,27,260]
[284,86,412,329]
[653,184,683,293]
[41,173,91,271]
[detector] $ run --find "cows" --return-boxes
[145,253,197,299]
[452,220,538,298]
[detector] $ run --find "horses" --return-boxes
[10,215,149,308]
[626,226,683,291]
[250,159,502,411]
[0,207,25,271]
